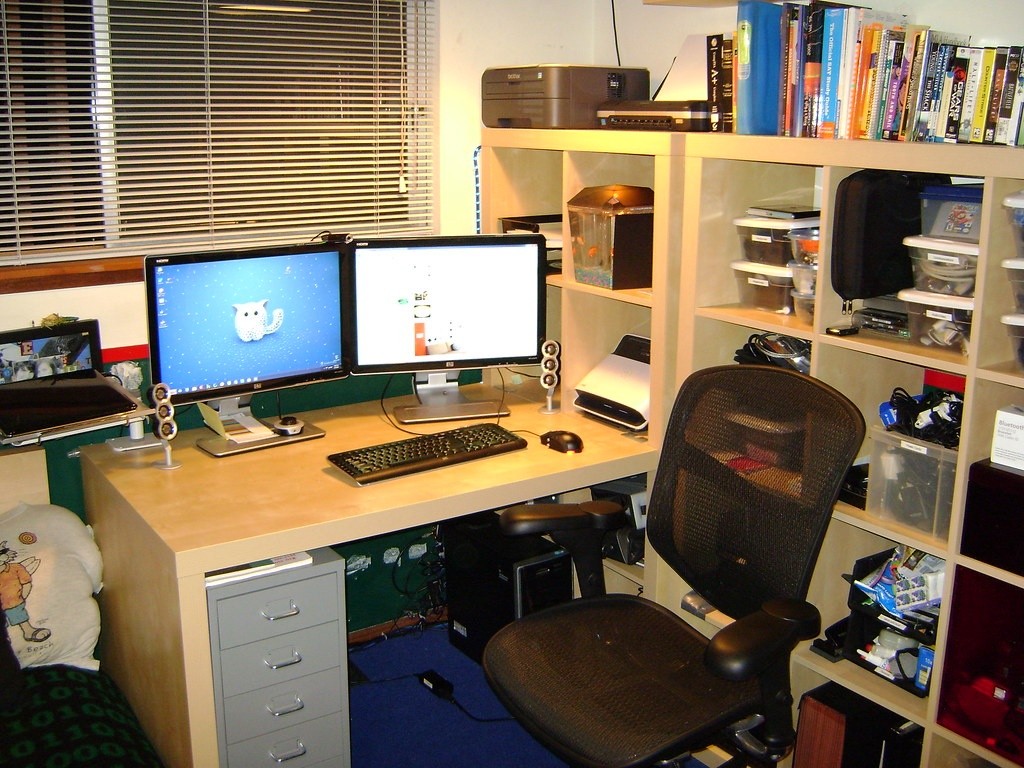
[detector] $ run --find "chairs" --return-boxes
[479,365,868,768]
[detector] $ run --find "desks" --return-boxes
[8,376,157,453]
[79,378,661,768]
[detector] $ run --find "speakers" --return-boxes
[538,340,561,414]
[146,383,182,470]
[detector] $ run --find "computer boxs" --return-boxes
[440,510,573,665]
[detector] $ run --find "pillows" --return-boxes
[0,503,106,671]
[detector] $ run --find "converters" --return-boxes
[418,669,453,700]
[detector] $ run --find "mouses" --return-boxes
[539,431,585,454]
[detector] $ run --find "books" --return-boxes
[706,0,1024,147]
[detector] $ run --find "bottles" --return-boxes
[866,645,896,659]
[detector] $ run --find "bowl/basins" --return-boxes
[790,289,815,326]
[787,260,819,294]
[784,230,819,264]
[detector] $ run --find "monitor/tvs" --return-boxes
[145,233,546,457]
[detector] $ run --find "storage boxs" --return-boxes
[483,64,650,129]
[497,212,562,274]
[865,427,958,547]
[730,215,822,267]
[902,234,980,297]
[567,182,654,291]
[1000,186,1024,368]
[917,186,984,244]
[896,287,971,359]
[730,259,795,312]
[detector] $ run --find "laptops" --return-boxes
[0,367,138,438]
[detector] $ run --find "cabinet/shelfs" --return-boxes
[205,548,352,768]
[662,106,1024,768]
[476,130,686,613]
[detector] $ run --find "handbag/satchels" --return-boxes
[831,168,954,315]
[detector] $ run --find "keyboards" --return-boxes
[328,422,528,486]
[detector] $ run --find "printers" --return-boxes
[481,66,651,131]
